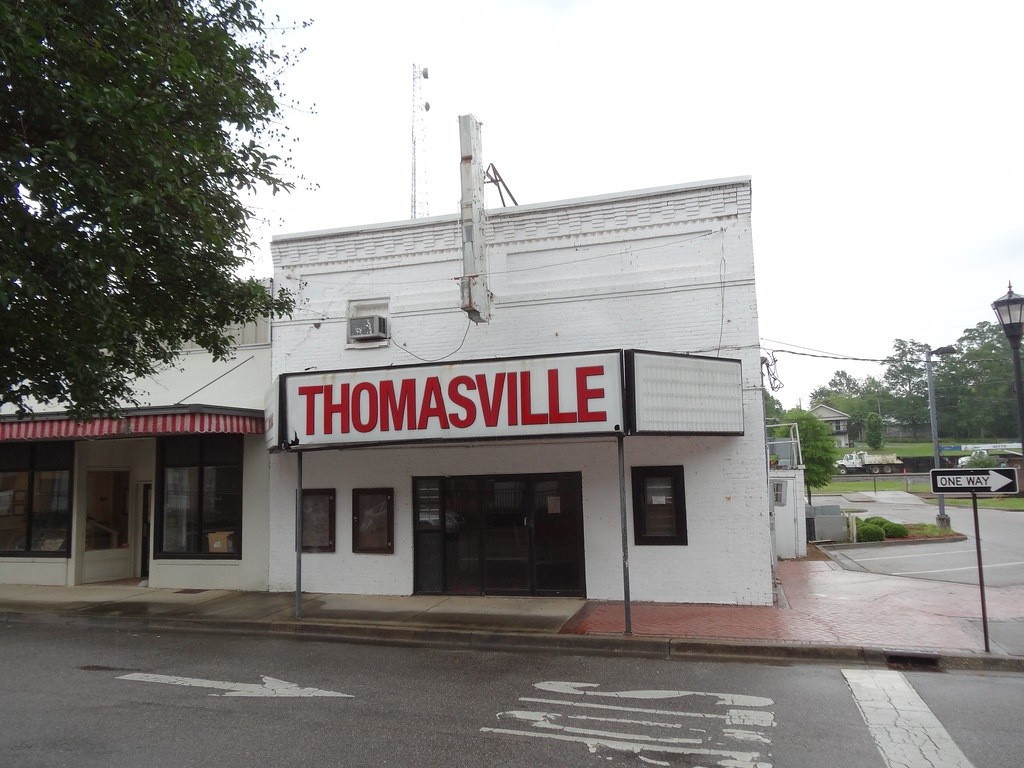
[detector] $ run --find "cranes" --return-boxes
[411,64,430,218]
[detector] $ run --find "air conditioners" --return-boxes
[348,315,387,341]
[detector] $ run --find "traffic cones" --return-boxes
[903,469,906,475]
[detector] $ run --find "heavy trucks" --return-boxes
[833,451,903,475]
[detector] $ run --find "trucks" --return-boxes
[957,450,988,466]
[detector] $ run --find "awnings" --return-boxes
[0,405,265,441]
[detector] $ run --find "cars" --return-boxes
[364,502,465,533]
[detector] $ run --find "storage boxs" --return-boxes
[208,532,234,552]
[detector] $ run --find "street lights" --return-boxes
[926,347,957,529]
[991,282,1024,456]
